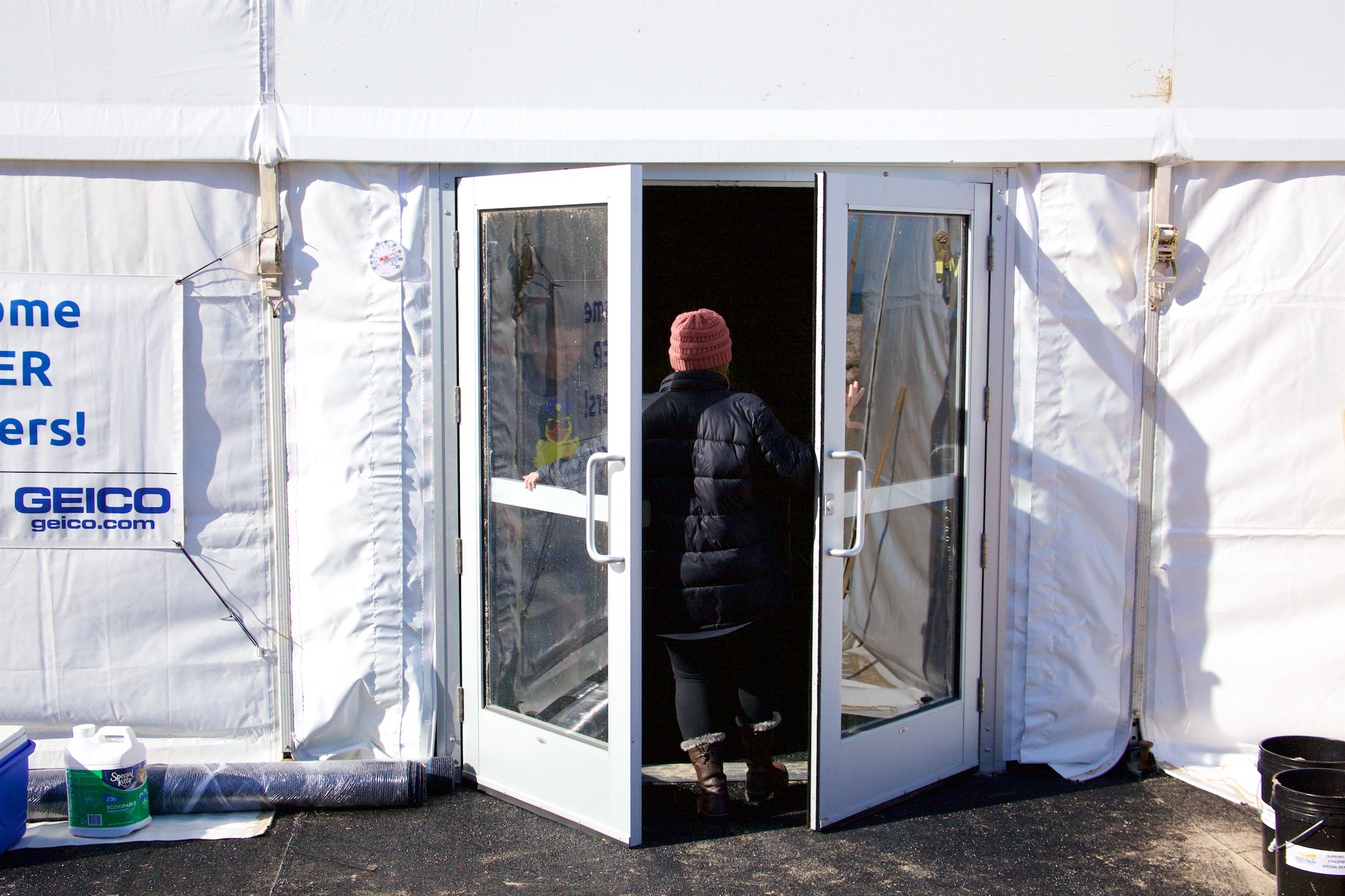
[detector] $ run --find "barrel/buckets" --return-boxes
[1258,735,1345,874]
[1266,768,1345,896]
[63,723,152,839]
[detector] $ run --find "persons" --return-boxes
[642,306,867,818]
[485,298,606,713]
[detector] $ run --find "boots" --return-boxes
[679,732,731,820]
[734,711,788,802]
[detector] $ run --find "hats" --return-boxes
[668,308,733,371]
